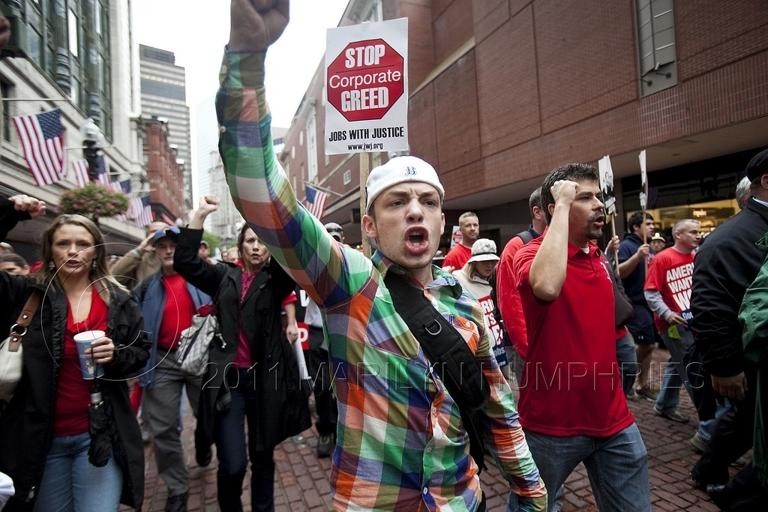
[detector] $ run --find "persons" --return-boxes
[0,1,768,511]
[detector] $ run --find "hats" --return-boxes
[746,149,767,181]
[364,155,444,215]
[468,238,501,264]
[735,177,751,205]
[324,222,344,243]
[151,225,181,245]
[650,228,667,242]
[200,240,209,249]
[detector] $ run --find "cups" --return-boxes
[73,329,110,380]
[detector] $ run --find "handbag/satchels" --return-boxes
[174,267,233,378]
[0,289,42,403]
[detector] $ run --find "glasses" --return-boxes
[326,228,342,232]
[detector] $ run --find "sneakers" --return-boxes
[689,431,746,467]
[653,405,689,423]
[316,431,334,458]
[139,417,151,445]
[635,384,657,401]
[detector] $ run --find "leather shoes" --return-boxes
[163,492,189,512]
[196,442,212,467]
